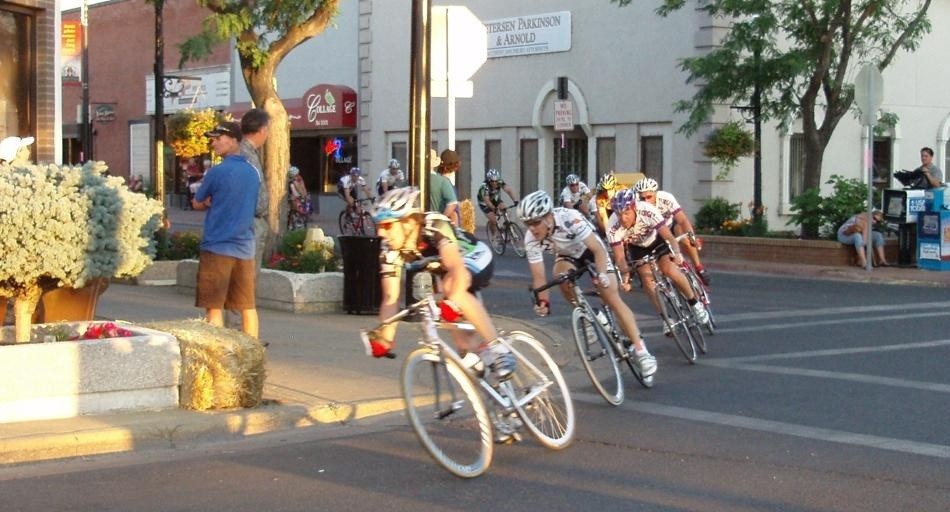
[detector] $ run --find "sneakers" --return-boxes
[662,318,675,334]
[494,411,524,443]
[581,323,599,344]
[879,260,890,266]
[637,353,658,377]
[699,268,713,286]
[690,301,709,325]
[479,349,517,376]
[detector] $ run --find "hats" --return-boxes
[432,150,441,167]
[0,137,34,163]
[205,122,241,142]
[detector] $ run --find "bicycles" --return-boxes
[676,230,718,335]
[355,295,576,481]
[588,212,644,290]
[484,203,527,259]
[338,197,376,236]
[528,259,655,407]
[288,192,314,232]
[614,241,707,365]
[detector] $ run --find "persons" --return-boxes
[195,159,211,182]
[337,168,368,229]
[476,171,518,242]
[286,167,311,218]
[375,159,403,197]
[0,135,37,165]
[910,146,943,189]
[516,170,710,378]
[836,209,891,268]
[366,183,524,448]
[231,107,271,348]
[190,120,262,344]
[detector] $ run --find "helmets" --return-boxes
[389,159,401,168]
[517,191,551,221]
[487,169,500,180]
[566,174,578,184]
[611,189,635,215]
[636,178,658,192]
[350,167,361,175]
[372,186,422,223]
[600,175,617,190]
[288,167,300,177]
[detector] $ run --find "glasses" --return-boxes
[873,216,880,224]
[524,221,542,227]
[640,195,652,200]
[374,221,398,230]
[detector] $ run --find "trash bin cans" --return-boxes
[338,236,381,314]
